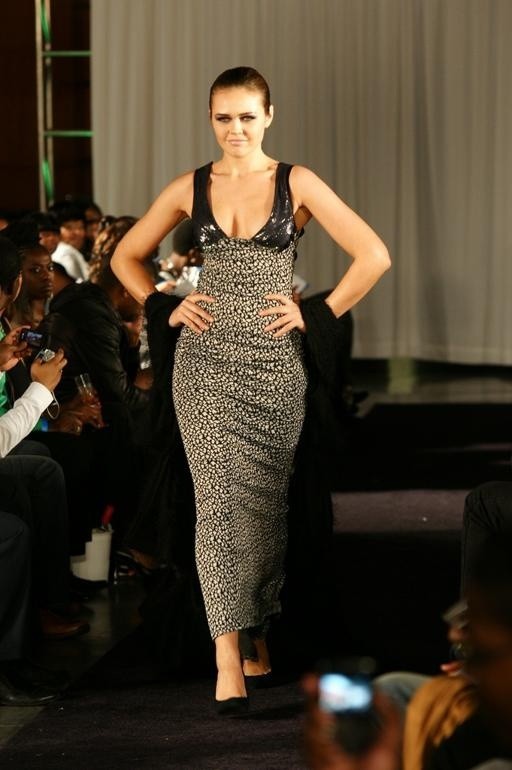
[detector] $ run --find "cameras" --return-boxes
[19,328,47,350]
[35,350,58,366]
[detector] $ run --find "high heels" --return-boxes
[212,633,277,715]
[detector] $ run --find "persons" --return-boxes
[301,488,511,768]
[1,199,307,712]
[110,66,391,715]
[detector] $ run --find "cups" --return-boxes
[73,372,106,430]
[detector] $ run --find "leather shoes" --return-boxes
[0,674,58,705]
[38,611,91,640]
